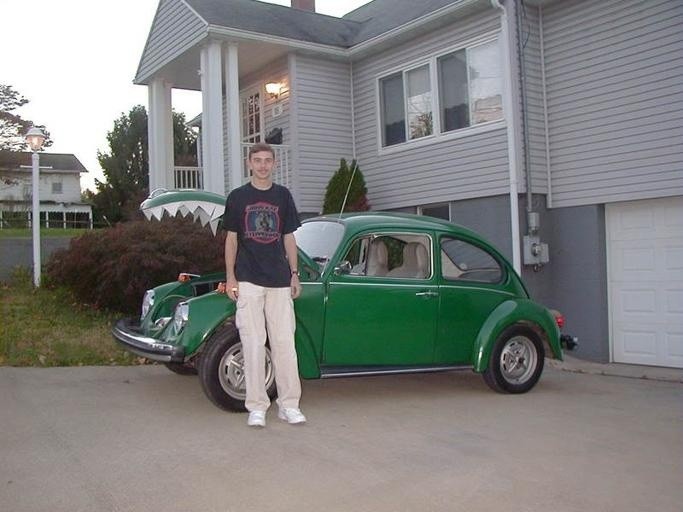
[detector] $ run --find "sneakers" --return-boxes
[278,408,306,424]
[248,411,266,427]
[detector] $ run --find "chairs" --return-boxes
[353,239,388,275]
[387,242,428,279]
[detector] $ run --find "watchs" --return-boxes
[290,269,302,277]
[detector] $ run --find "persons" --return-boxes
[226,141,307,429]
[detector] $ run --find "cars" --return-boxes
[114,187,578,412]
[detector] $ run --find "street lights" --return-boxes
[25,128,42,288]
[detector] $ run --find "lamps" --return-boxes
[265,82,279,98]
